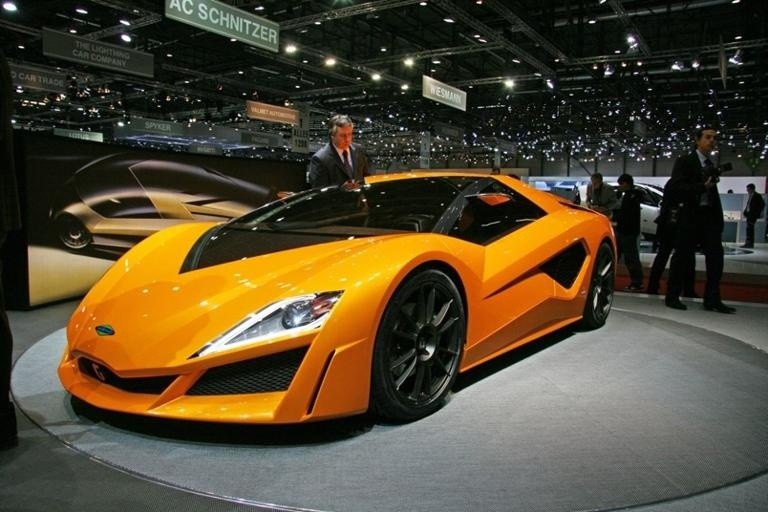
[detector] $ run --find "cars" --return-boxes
[575,178,664,240]
[528,179,553,192]
[553,176,589,189]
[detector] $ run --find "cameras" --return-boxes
[713,162,734,183]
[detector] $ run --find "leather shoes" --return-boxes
[682,290,701,298]
[665,296,687,310]
[647,288,660,295]
[704,301,736,313]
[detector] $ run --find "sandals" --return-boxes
[625,284,645,290]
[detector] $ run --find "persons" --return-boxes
[1,49,23,456]
[614,174,647,293]
[740,183,765,248]
[646,173,699,298]
[310,113,368,190]
[665,126,737,313]
[584,173,619,223]
[491,167,500,175]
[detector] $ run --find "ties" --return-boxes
[342,150,353,178]
[706,159,712,167]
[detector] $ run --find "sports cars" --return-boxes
[46,164,621,438]
[44,148,293,259]
